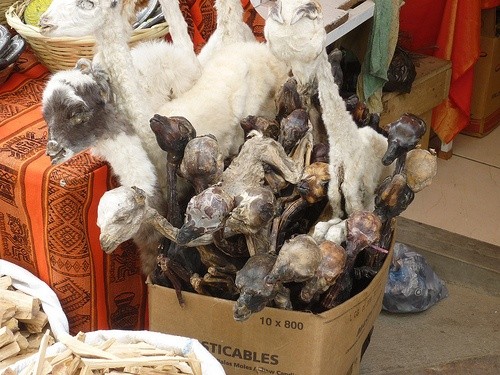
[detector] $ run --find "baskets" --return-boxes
[4,1,170,73]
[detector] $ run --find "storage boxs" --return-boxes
[4,0,170,75]
[459,7,500,139]
[144,214,399,375]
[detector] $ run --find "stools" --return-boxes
[378,53,453,150]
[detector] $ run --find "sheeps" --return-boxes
[39,0,448,322]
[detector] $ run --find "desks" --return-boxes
[0,0,407,337]
